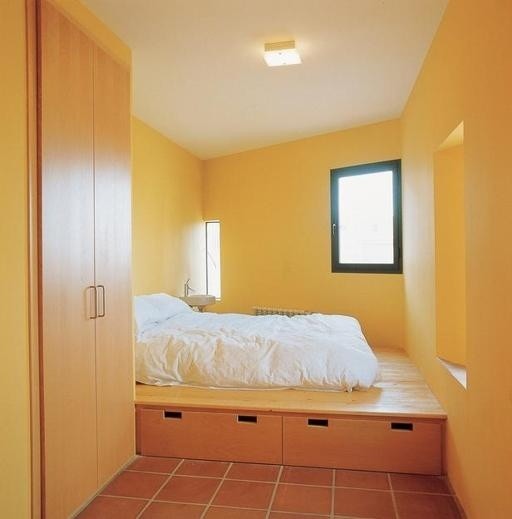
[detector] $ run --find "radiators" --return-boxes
[250,304,308,317]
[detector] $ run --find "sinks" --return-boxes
[178,295,215,306]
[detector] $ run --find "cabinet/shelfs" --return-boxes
[38,0,135,519]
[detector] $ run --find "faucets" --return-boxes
[183,278,195,296]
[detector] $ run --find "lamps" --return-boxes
[187,293,217,313]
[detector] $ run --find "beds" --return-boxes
[136,292,448,477]
